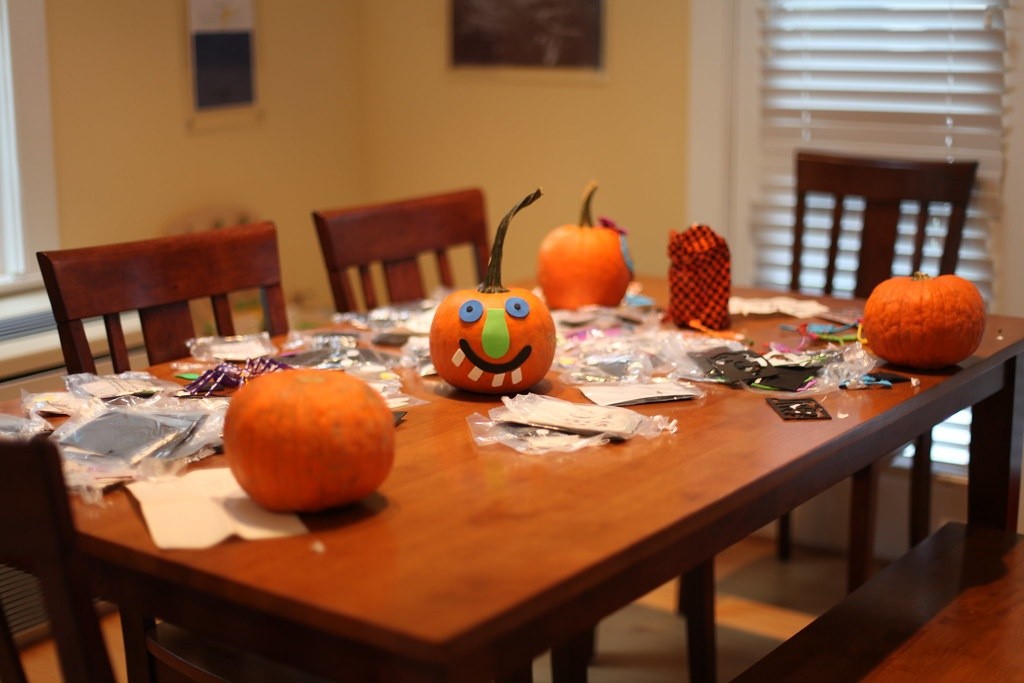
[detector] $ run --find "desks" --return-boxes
[727,519,1024,683]
[0,274,1024,683]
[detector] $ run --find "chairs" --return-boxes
[37,222,534,683]
[311,188,493,314]
[0,435,120,683]
[674,147,980,621]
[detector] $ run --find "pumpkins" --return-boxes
[225,369,396,511]
[861,272,984,366]
[430,187,556,393]
[538,183,634,308]
